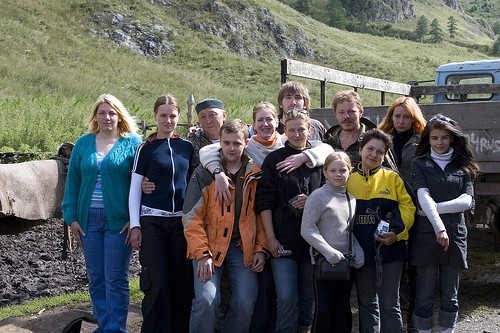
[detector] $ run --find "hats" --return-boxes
[195,99,224,114]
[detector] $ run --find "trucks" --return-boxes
[276,59,500,253]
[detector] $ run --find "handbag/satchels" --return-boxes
[311,251,354,283]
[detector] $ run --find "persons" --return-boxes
[60,81,481,333]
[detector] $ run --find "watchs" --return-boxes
[212,168,224,177]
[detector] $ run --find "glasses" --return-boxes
[428,116,450,122]
[286,108,308,115]
